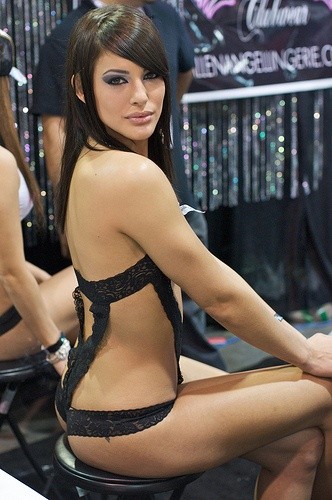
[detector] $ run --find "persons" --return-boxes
[0,31,80,380]
[30,0,225,376]
[52,5,332,500]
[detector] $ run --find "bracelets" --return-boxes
[43,333,70,364]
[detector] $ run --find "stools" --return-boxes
[43,432,206,500]
[0,348,68,483]
